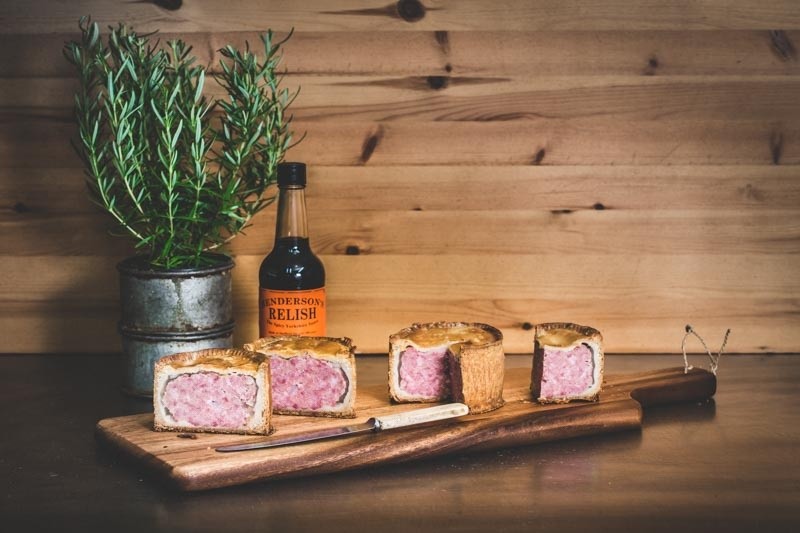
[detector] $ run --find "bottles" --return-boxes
[258,162,325,339]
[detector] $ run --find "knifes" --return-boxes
[215,403,470,453]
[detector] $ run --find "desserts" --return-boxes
[243,335,360,419]
[389,319,508,414]
[530,322,605,404]
[152,348,274,435]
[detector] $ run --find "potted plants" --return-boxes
[67,11,307,400]
